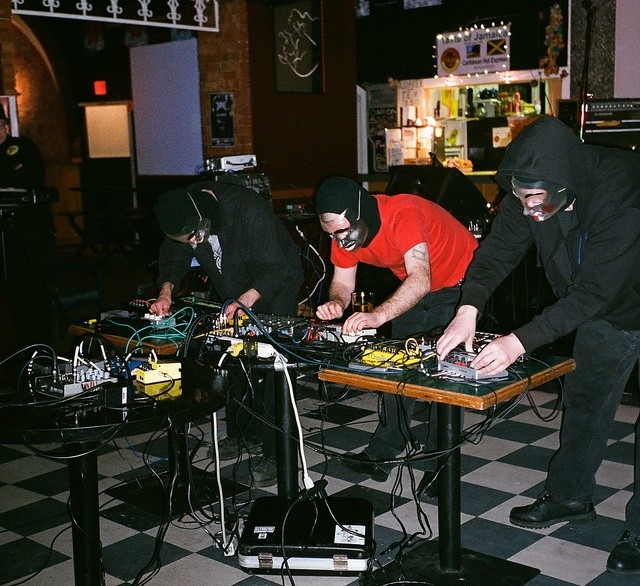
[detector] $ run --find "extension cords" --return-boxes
[222,518,245,556]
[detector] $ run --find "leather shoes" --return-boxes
[247,453,278,488]
[207,436,263,461]
[416,470,440,499]
[340,452,391,482]
[509,490,597,529]
[606,528,640,576]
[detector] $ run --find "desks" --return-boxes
[315,353,575,581]
[66,296,243,502]
[0,359,235,586]
[196,323,375,558]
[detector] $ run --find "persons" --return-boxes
[434,112,640,576]
[311,173,483,504]
[148,177,305,487]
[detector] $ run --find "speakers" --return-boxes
[436,24,511,75]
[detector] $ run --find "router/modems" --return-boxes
[237,497,375,571]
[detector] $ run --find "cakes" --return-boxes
[442,152,474,182]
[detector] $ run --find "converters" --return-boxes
[100,384,134,406]
